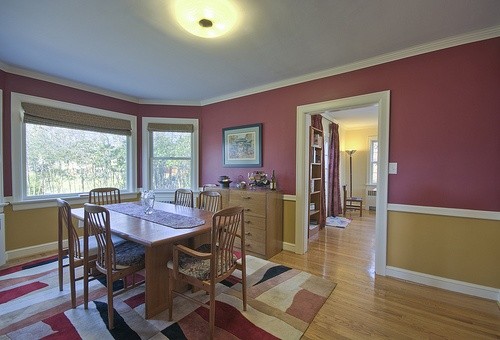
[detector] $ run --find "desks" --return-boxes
[67,197,241,320]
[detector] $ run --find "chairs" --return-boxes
[166,205,247,340]
[81,203,146,329]
[343,184,363,217]
[88,187,122,233]
[197,191,221,215]
[56,197,126,309]
[174,188,194,209]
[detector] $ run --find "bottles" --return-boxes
[270,170,276,190]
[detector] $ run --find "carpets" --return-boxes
[0,248,341,340]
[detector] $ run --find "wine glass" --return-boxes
[248,173,256,190]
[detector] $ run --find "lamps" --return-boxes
[173,1,242,40]
[345,149,358,211]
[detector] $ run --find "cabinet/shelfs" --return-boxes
[308,125,324,238]
[203,184,284,260]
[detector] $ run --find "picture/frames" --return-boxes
[221,123,263,168]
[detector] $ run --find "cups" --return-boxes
[141,193,155,214]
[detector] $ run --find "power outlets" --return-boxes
[78,220,84,228]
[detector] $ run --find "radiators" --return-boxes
[365,188,377,211]
